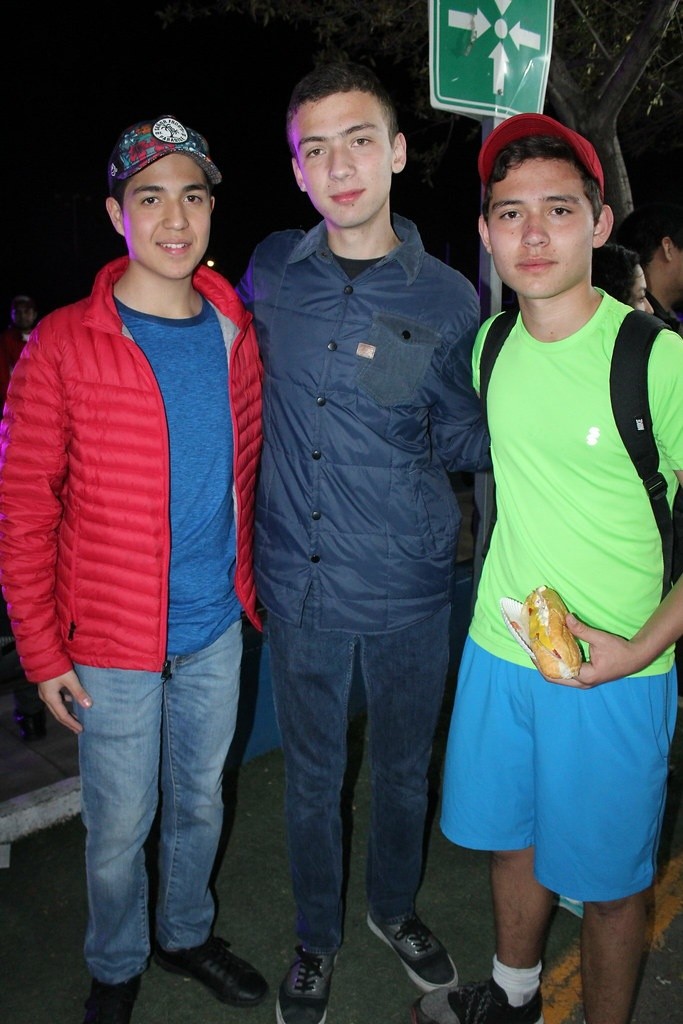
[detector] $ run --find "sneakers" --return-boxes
[83,975,141,1024]
[275,945,338,1024]
[410,977,544,1024]
[154,936,269,1006]
[366,911,459,993]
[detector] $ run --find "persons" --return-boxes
[233,65,492,1024]
[0,116,269,1024]
[414,113,683,1024]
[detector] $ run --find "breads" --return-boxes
[524,586,583,680]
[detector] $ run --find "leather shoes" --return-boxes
[13,707,47,741]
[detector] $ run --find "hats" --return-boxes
[107,115,222,195]
[478,113,605,202]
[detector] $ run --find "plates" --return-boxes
[500,597,536,659]
